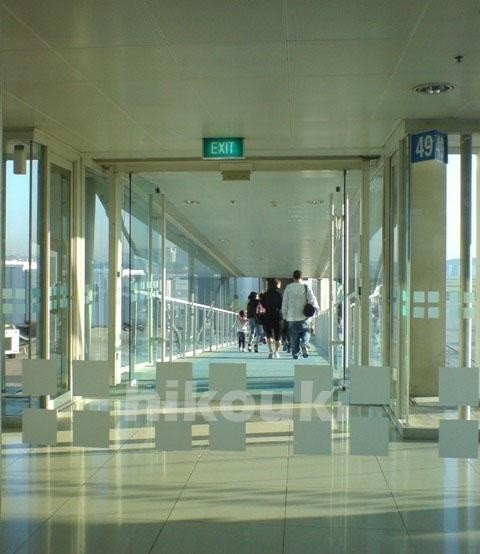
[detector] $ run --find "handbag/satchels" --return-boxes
[304,303,315,316]
[255,312,265,324]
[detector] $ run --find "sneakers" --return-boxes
[239,344,258,352]
[269,342,308,359]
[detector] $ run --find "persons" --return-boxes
[282,269,318,357]
[261,278,283,359]
[230,277,311,352]
